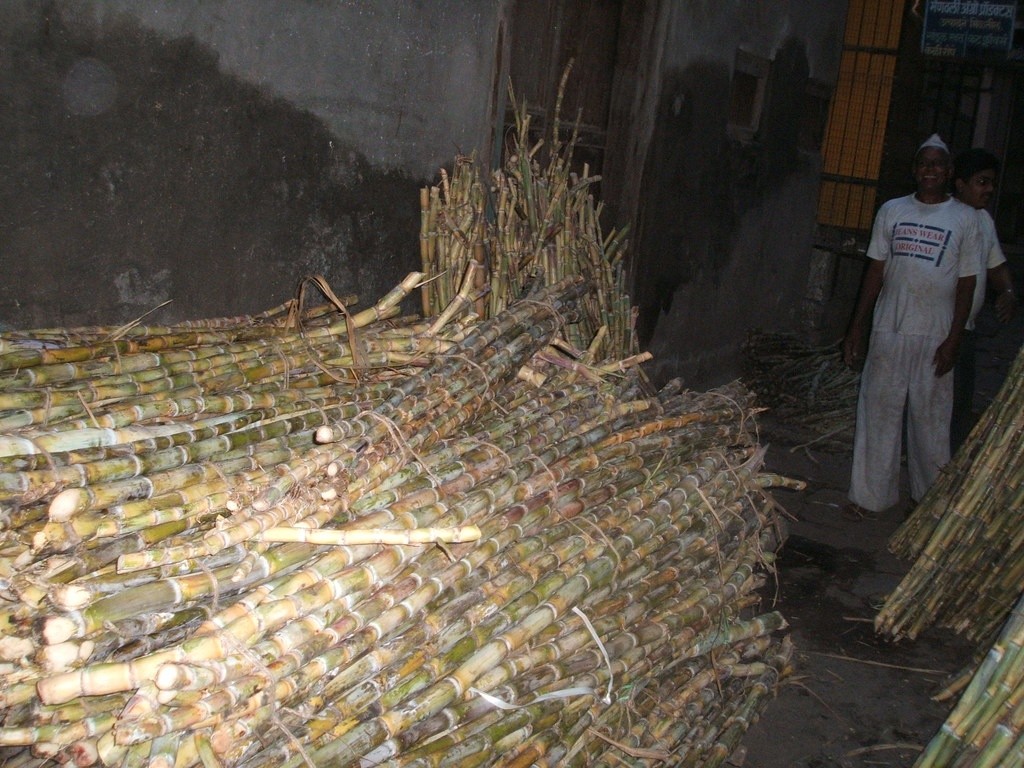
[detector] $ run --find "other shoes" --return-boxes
[842,502,873,521]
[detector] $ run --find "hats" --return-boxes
[914,133,950,157]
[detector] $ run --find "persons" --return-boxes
[842,131,980,525]
[950,147,1016,446]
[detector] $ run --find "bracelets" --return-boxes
[998,288,1016,302]
